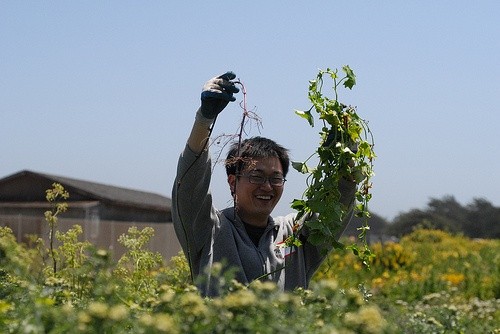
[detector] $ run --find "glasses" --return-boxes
[237,171,287,187]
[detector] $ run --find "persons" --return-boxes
[171,71,360,299]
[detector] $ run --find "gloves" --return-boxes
[322,124,358,162]
[195,70,240,124]
